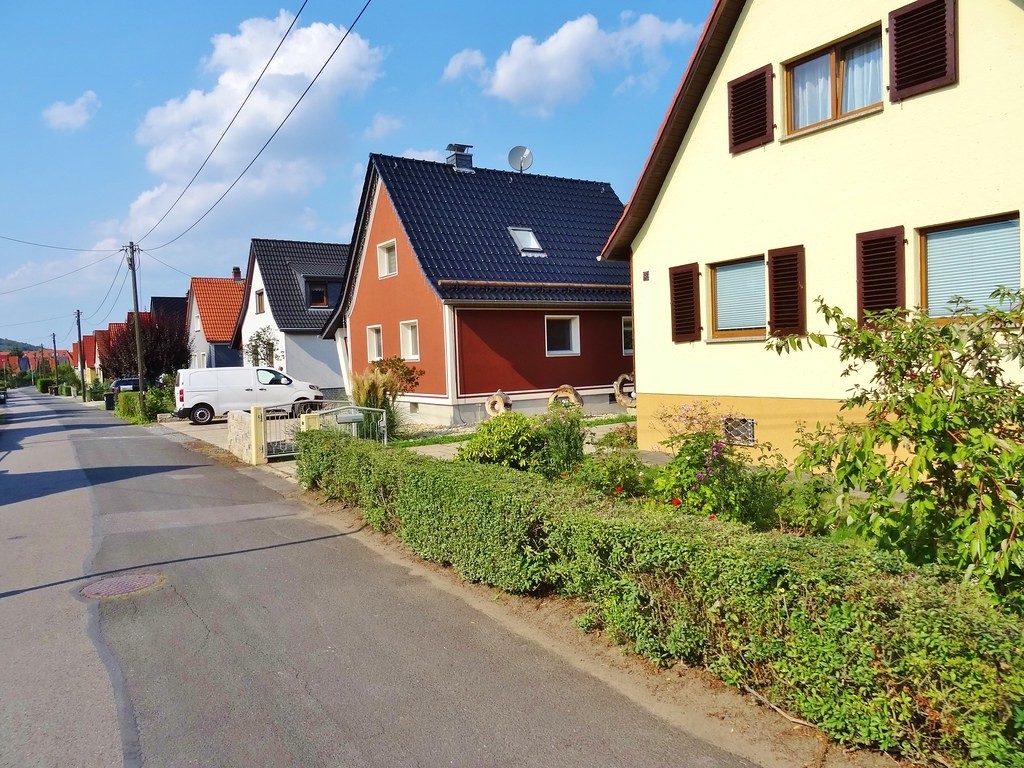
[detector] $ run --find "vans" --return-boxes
[170,366,324,425]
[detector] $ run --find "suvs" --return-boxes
[110,378,164,392]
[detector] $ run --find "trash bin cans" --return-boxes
[53,386,59,396]
[103,393,116,411]
[0,391,6,404]
[48,386,53,395]
